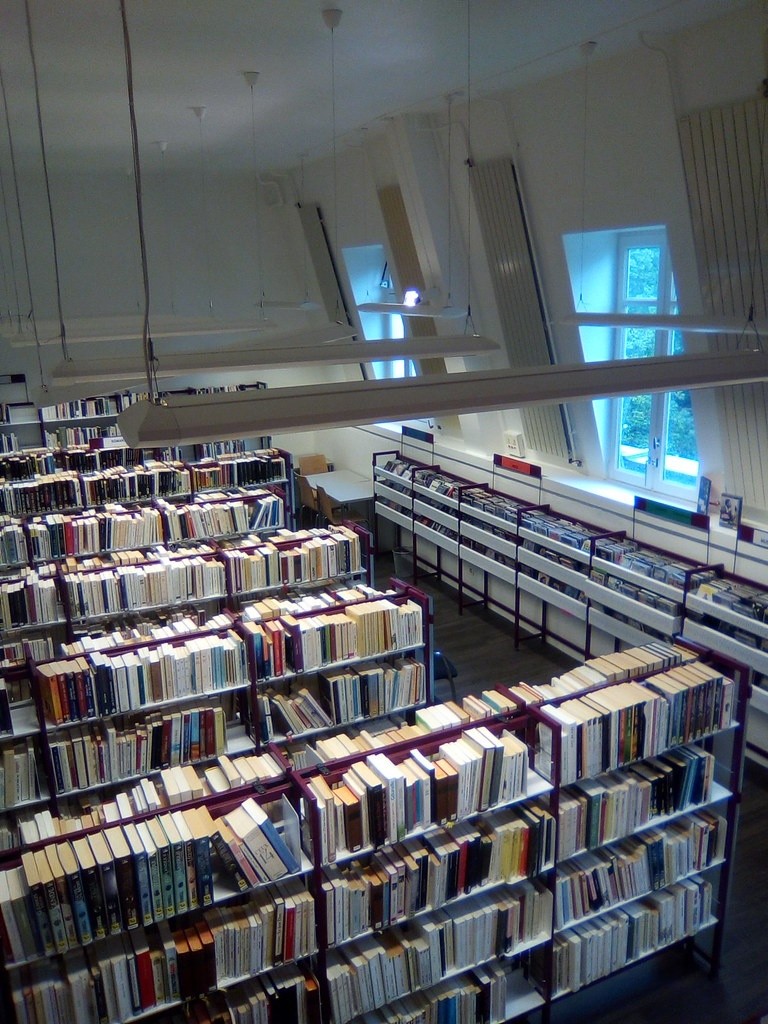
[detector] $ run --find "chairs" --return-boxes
[293,454,370,531]
[434,651,457,703]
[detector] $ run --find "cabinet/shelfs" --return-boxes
[0,576,435,816]
[0,631,754,1024]
[371,450,768,769]
[0,483,287,573]
[0,520,376,641]
[0,447,297,532]
[0,381,272,461]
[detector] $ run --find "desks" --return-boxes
[293,456,336,511]
[305,468,383,525]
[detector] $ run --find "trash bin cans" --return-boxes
[392,546,414,584]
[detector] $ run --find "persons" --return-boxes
[721,499,736,526]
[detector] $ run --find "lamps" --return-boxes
[356,102,467,318]
[0,27,358,409]
[253,159,320,312]
[23,0,501,388]
[0,84,278,349]
[116,0,768,449]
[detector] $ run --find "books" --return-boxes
[0,381,768,1024]
